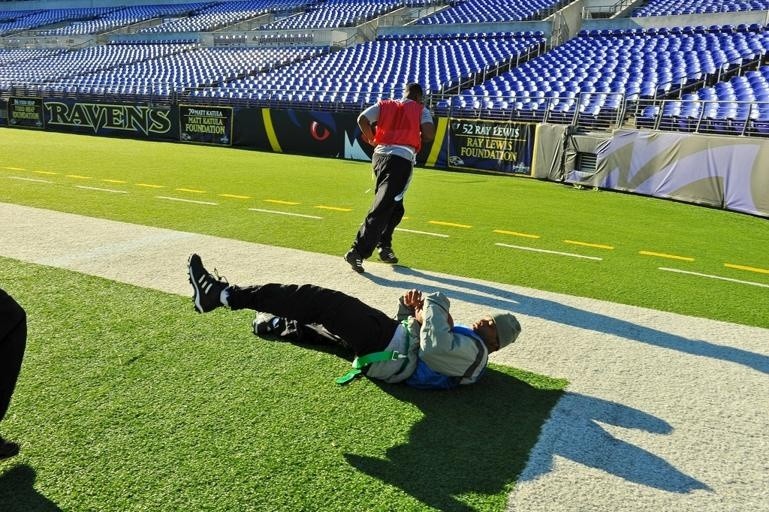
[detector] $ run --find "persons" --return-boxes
[187,253,521,389]
[344,83,434,273]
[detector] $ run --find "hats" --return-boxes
[489,312,521,349]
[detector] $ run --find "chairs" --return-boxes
[2,2,769,140]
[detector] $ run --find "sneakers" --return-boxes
[344,248,364,273]
[378,248,398,263]
[187,253,229,314]
[252,310,275,334]
[0,436,19,459]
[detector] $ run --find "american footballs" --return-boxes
[414,302,454,332]
